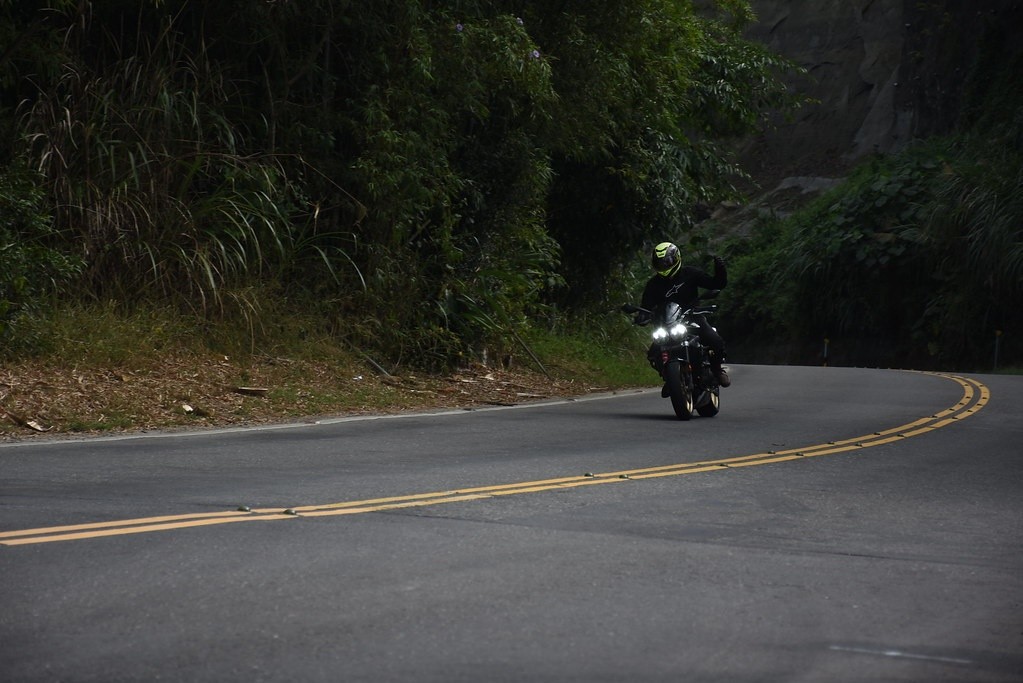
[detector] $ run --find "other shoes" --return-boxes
[715,368,731,387]
[661,381,671,398]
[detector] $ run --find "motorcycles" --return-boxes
[621,289,727,420]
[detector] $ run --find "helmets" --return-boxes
[652,242,682,280]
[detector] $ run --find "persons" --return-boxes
[633,242,731,399]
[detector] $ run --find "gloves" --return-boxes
[714,256,727,273]
[636,311,652,326]
